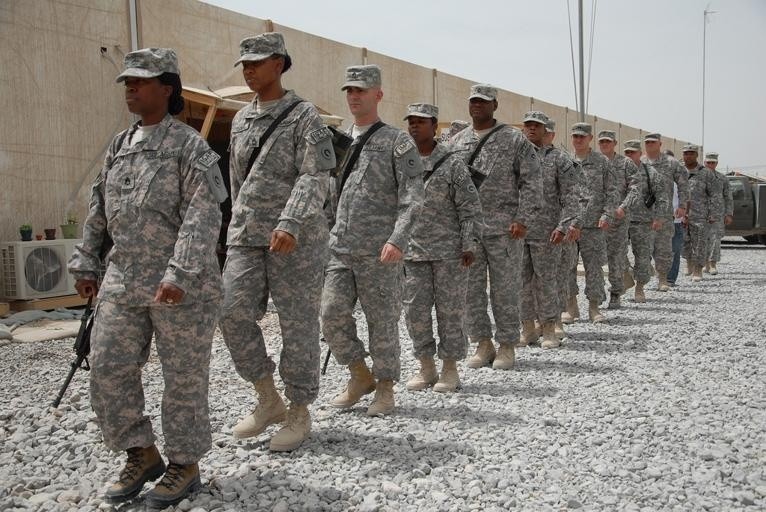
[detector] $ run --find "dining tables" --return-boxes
[18,224,32,241]
[58,207,82,240]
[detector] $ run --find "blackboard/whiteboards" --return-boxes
[722,171,766,244]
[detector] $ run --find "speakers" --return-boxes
[368,379,395,416]
[234,375,287,438]
[609,259,718,309]
[468,339,495,368]
[269,402,311,451]
[493,343,514,370]
[589,301,604,323]
[433,360,460,392]
[332,361,376,408]
[517,297,580,350]
[406,355,439,390]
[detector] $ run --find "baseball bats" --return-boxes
[52,294,93,408]
[320,338,331,374]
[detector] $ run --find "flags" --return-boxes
[35,235,41,240]
[43,228,56,240]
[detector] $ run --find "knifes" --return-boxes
[0,238,81,302]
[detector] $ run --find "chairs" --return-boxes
[104,444,166,503]
[146,460,201,509]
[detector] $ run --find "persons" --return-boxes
[65,45,230,507]
[217,30,339,454]
[640,132,691,292]
[561,122,617,325]
[703,152,733,275]
[321,60,428,417]
[608,139,669,304]
[534,116,590,339]
[441,84,545,373]
[662,150,684,287]
[447,118,470,136]
[402,98,484,398]
[681,144,719,282]
[598,130,642,309]
[520,112,580,348]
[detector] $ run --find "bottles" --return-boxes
[706,153,718,162]
[523,111,555,133]
[234,33,286,66]
[644,134,660,141]
[598,131,615,141]
[624,140,640,152]
[571,123,591,137]
[115,48,178,83]
[402,103,438,121]
[683,145,698,154]
[468,85,495,101]
[341,65,382,90]
[449,120,469,136]
